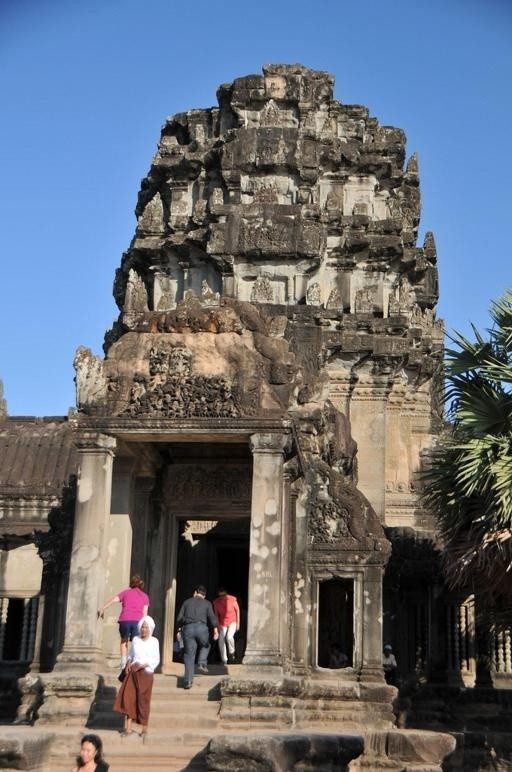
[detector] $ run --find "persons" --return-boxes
[100,573,150,666]
[381,644,399,684]
[176,584,220,689]
[72,734,112,770]
[212,588,243,663]
[328,640,349,667]
[114,613,162,736]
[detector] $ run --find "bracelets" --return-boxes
[177,630,181,632]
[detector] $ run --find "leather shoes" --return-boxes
[197,663,209,674]
[184,681,192,689]
